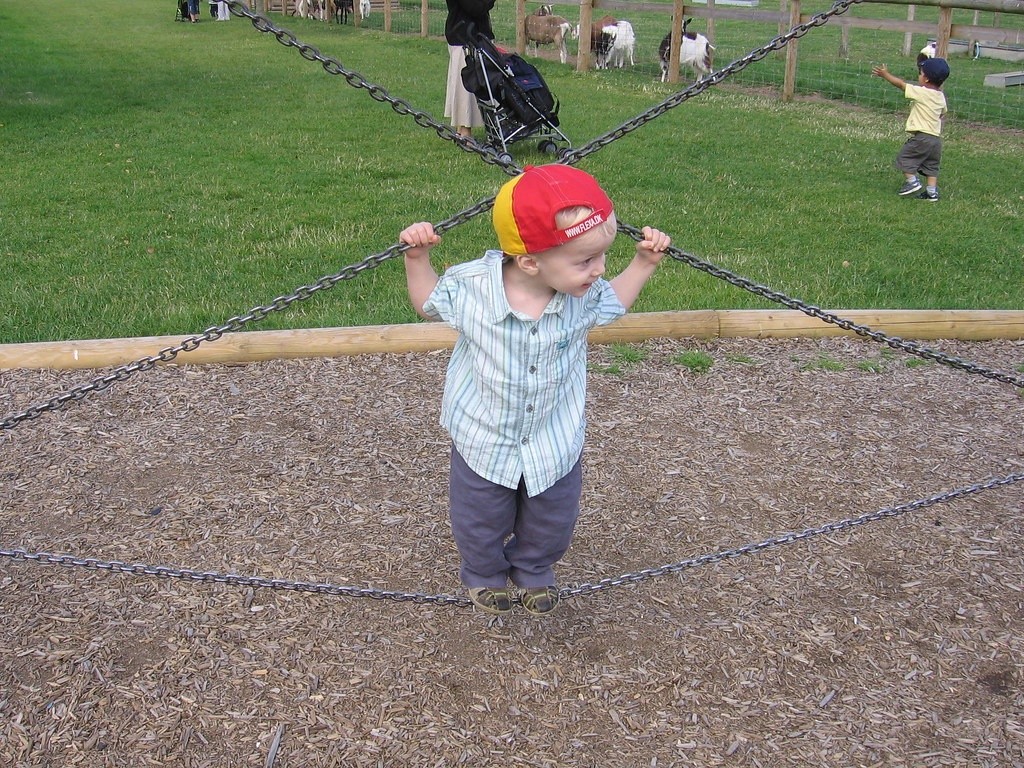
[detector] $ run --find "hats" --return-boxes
[915,53,951,85]
[491,164,614,256]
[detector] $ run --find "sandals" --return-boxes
[468,585,514,614]
[520,585,562,617]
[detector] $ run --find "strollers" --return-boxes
[173,0,190,22]
[453,18,577,168]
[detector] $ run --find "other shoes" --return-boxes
[216,17,230,21]
[191,18,200,24]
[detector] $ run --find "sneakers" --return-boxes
[898,178,923,197]
[916,190,939,202]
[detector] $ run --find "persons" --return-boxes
[215,0,230,21]
[443,0,496,141]
[870,57,951,201]
[188,0,200,23]
[399,164,670,615]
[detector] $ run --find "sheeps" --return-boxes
[523,4,573,64]
[291,0,370,25]
[917,40,938,71]
[572,15,636,70]
[659,15,716,83]
